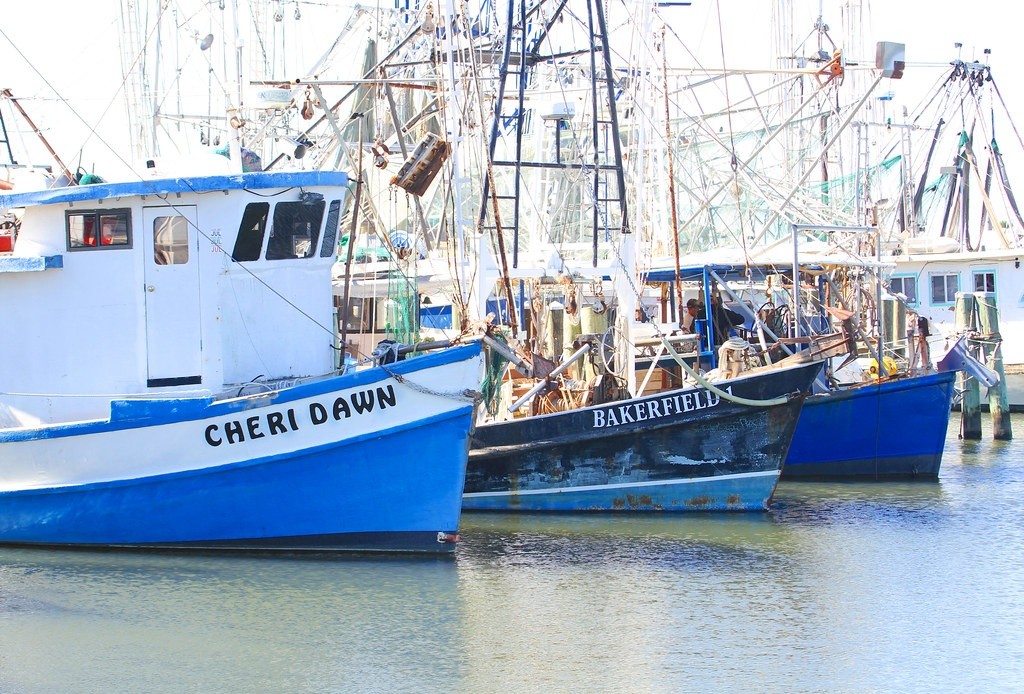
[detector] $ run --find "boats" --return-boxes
[1,160,484,559]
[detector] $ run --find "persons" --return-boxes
[683,286,746,347]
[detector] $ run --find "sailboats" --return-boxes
[121,0,1000,513]
